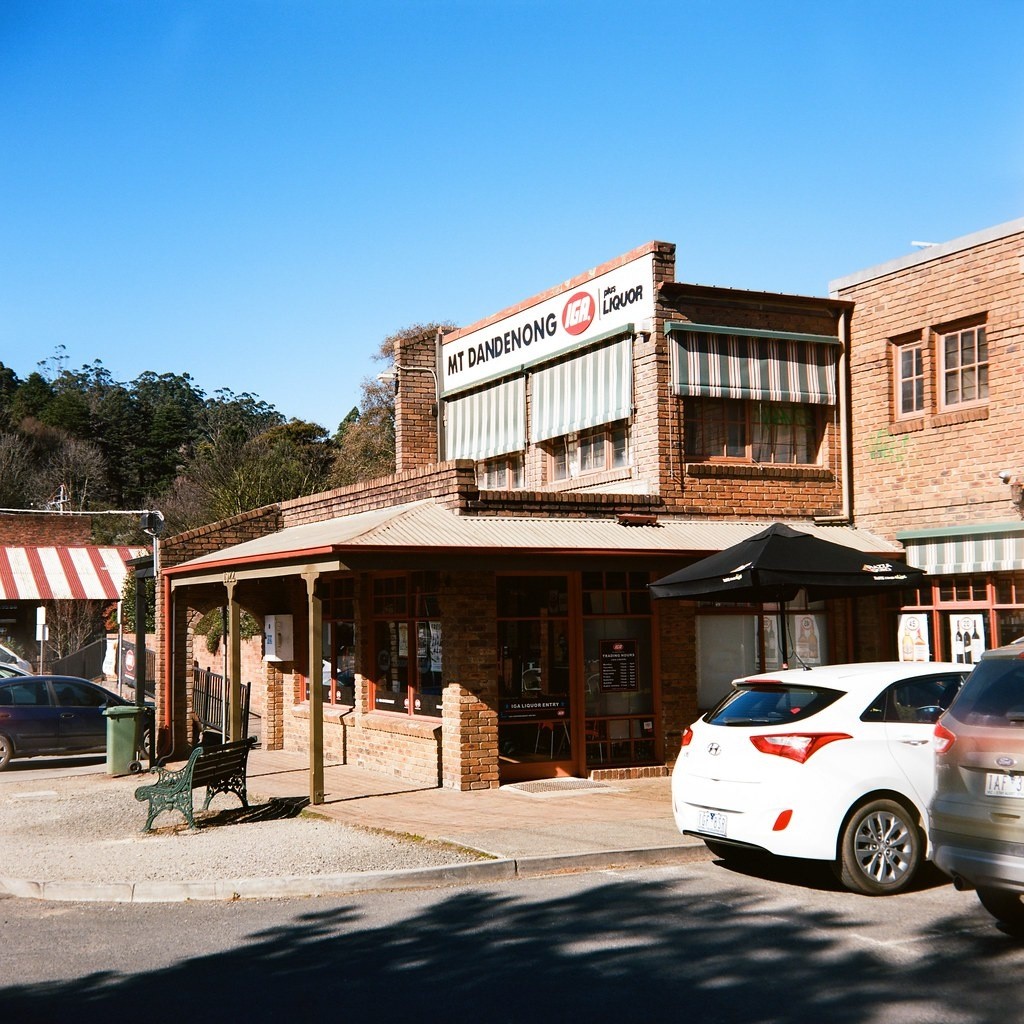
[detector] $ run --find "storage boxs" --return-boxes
[265,614,293,661]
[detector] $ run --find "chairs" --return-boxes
[916,706,945,723]
[585,720,604,764]
[535,722,570,759]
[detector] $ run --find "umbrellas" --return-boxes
[652,523,931,672]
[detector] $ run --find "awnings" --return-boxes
[0,544,157,601]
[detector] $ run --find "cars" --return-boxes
[0,662,33,679]
[1,643,32,672]
[927,636,1024,927]
[668,661,977,898]
[0,675,155,771]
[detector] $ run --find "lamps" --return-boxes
[140,512,162,537]
[378,365,398,387]
[1011,476,1023,504]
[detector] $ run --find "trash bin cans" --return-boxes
[101,705,157,777]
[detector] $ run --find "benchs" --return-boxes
[136,735,258,832]
[750,710,787,723]
